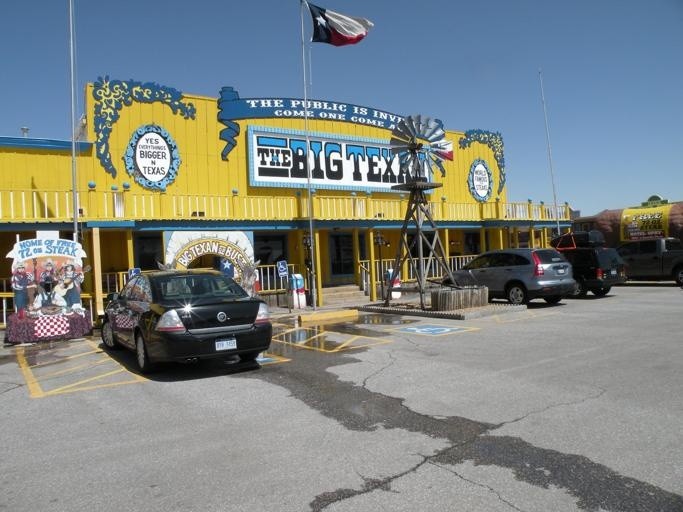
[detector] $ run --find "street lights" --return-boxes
[374,230,391,300]
[303,235,316,307]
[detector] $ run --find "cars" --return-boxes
[441,230,683,304]
[101,268,272,373]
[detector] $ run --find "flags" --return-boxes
[304,1,376,47]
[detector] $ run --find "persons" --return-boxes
[11,259,85,314]
